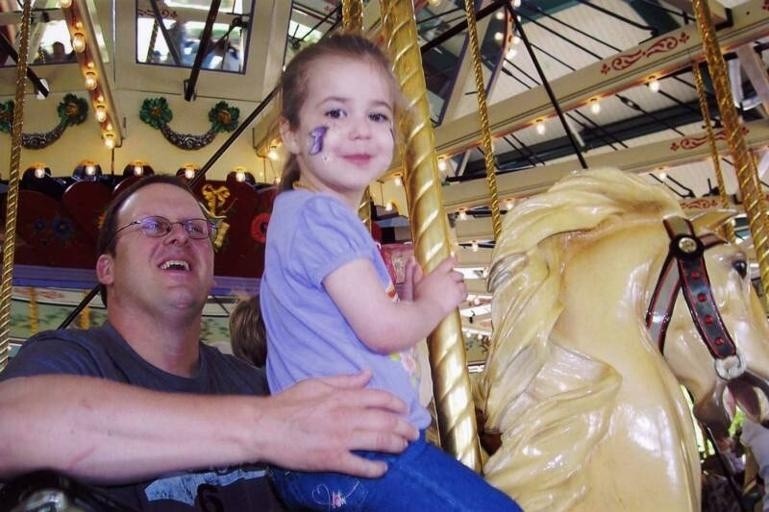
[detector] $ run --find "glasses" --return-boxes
[100,212,217,252]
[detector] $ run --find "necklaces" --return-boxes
[291,176,321,195]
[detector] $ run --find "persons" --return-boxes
[0,173,420,511]
[33,19,246,76]
[227,290,269,371]
[700,419,768,511]
[256,32,522,511]
